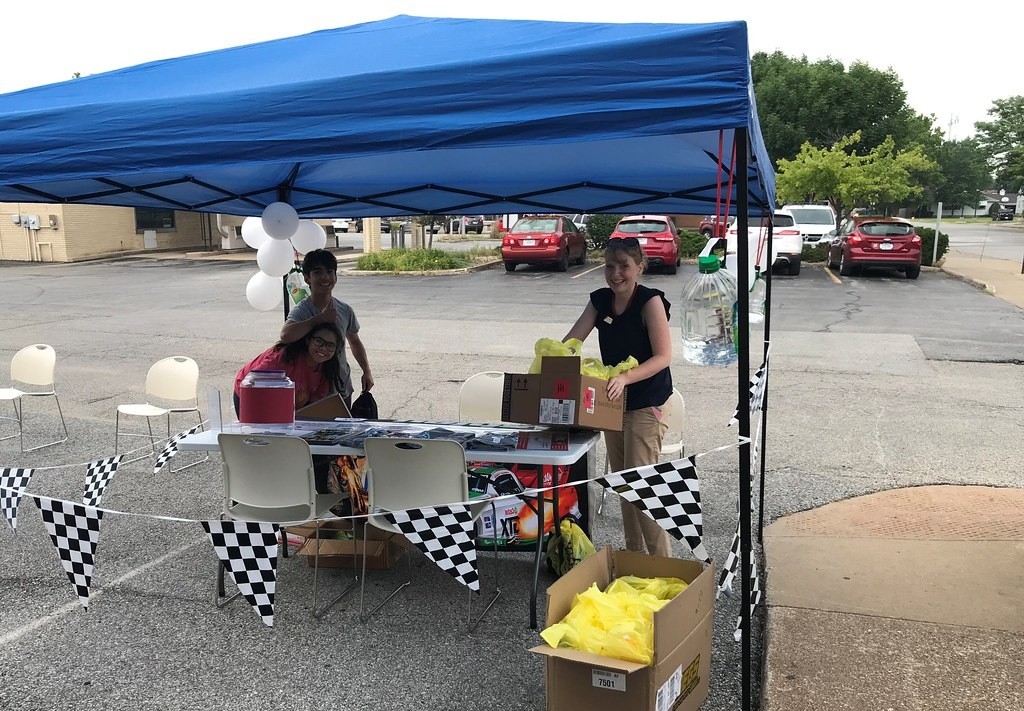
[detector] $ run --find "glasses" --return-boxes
[311,335,339,352]
[606,237,643,261]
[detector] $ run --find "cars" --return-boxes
[608,215,682,275]
[827,216,923,280]
[501,214,596,272]
[698,200,837,276]
[399,215,483,234]
[331,218,391,233]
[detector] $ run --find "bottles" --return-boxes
[679,254,739,367]
[285,269,312,306]
[239,369,296,437]
[749,270,767,324]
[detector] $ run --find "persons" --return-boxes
[282,248,374,412]
[234,319,344,496]
[559,237,675,558]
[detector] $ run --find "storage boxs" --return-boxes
[501,356,628,432]
[282,520,417,570]
[527,545,715,711]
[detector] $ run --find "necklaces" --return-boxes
[624,282,637,310]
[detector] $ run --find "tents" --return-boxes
[0,14,775,711]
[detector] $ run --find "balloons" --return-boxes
[246,271,285,311]
[748,231,777,273]
[290,219,327,255]
[262,202,300,240]
[719,255,755,293]
[242,216,273,249]
[257,239,295,277]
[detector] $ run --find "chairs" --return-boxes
[359,436,502,632]
[115,356,210,473]
[0,344,68,452]
[214,432,359,619]
[458,371,505,422]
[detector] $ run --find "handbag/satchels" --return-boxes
[546,515,583,580]
[547,520,597,577]
[350,390,379,418]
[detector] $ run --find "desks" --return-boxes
[176,421,602,629]
